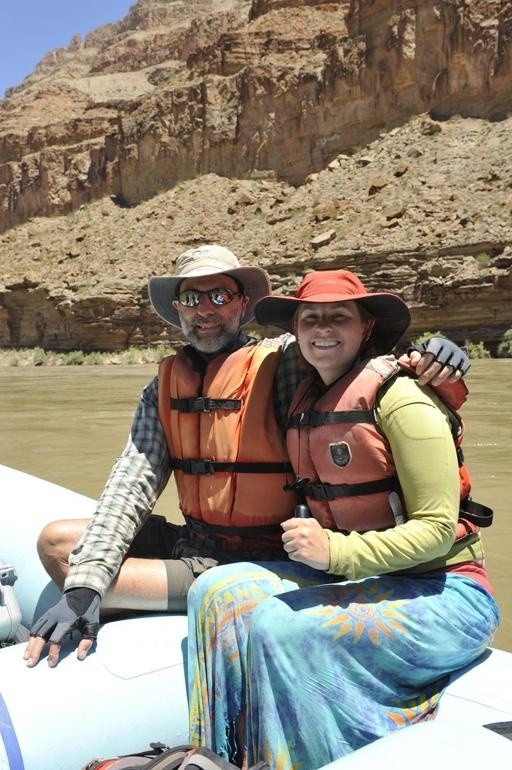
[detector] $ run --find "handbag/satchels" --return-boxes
[85,744,240,769]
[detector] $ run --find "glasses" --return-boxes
[174,286,246,308]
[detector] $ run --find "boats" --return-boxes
[0,462,512,770]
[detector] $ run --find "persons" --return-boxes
[23,244,473,670]
[181,264,504,770]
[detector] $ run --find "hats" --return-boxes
[147,245,272,330]
[253,269,411,357]
